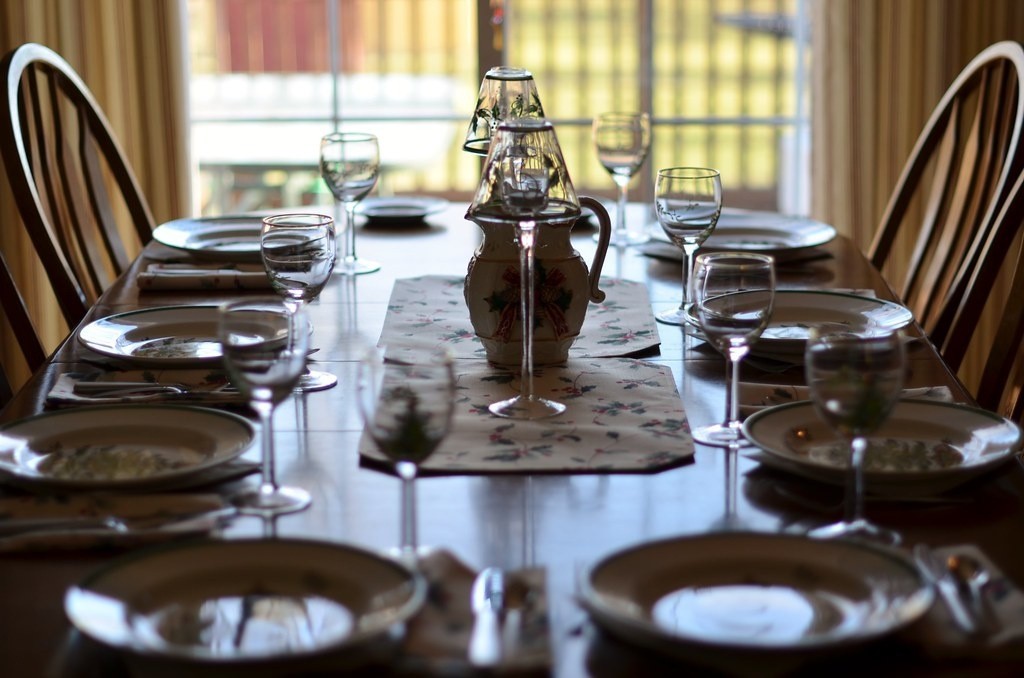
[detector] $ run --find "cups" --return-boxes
[461,69,551,155]
[473,121,582,233]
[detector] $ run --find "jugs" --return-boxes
[462,196,611,366]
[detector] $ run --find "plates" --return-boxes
[346,195,448,222]
[74,304,313,366]
[687,290,914,357]
[150,213,330,261]
[573,531,941,657]
[644,207,838,261]
[60,533,425,669]
[1,407,254,491]
[742,394,1022,495]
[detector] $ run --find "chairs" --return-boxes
[0,40,157,405]
[865,40,1024,466]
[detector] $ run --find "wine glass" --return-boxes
[216,297,314,519]
[261,211,339,392]
[591,111,650,246]
[312,131,382,275]
[354,341,454,584]
[694,246,761,449]
[803,324,904,549]
[654,167,723,325]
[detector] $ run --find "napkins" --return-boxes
[136,262,271,289]
[0,492,221,549]
[392,551,552,678]
[912,545,1024,658]
[45,367,246,408]
[0,202,1024,678]
[727,375,954,415]
